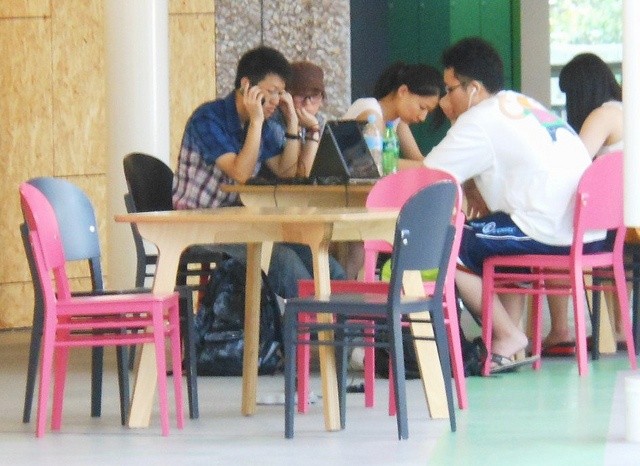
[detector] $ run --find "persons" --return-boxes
[542,51,640,355]
[171,46,347,367]
[420,36,608,376]
[266,61,365,281]
[343,62,444,170]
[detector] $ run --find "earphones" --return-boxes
[469,87,480,101]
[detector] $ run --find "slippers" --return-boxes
[587,336,630,353]
[491,351,540,374]
[527,341,576,356]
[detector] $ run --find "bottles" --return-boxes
[361,115,382,177]
[382,122,400,176]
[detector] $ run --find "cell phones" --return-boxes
[248,87,265,105]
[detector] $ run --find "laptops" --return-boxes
[248,122,326,184]
[327,120,382,183]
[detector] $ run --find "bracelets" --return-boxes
[305,135,319,144]
[306,127,320,134]
[284,131,302,140]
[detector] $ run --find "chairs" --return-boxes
[281,179,459,443]
[121,151,229,370]
[21,175,200,427]
[16,180,185,440]
[480,152,638,376]
[297,167,467,414]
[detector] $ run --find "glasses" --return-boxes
[289,92,322,104]
[446,82,468,95]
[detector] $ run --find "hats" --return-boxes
[284,62,325,94]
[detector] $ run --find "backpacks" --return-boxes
[193,259,285,376]
[375,257,488,378]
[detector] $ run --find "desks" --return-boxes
[113,205,449,431]
[221,178,617,354]
